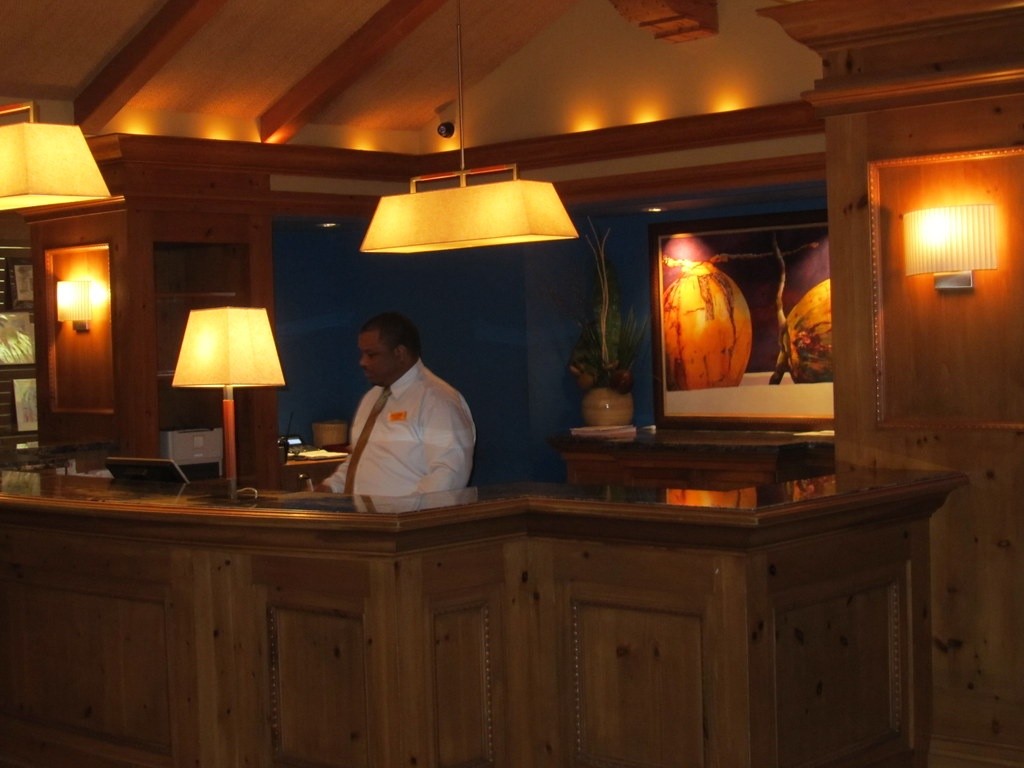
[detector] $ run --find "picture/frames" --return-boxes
[645,207,839,433]
[6,255,35,312]
[13,378,37,432]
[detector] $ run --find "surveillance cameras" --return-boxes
[434,101,457,139]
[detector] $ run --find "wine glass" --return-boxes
[292,443,301,460]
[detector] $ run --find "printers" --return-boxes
[160,425,226,481]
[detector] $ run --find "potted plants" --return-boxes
[562,213,652,426]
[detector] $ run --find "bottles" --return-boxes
[277,437,289,464]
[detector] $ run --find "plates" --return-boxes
[287,449,348,459]
[569,425,636,442]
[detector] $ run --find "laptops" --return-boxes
[104,456,190,484]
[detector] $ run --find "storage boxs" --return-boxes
[160,424,224,460]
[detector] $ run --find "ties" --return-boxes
[344,388,392,495]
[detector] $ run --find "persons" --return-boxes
[301,312,476,496]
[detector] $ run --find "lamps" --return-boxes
[897,200,998,300]
[55,278,95,332]
[0,100,116,212]
[357,0,581,256]
[172,302,287,507]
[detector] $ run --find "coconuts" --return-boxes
[660,260,753,389]
[781,277,830,383]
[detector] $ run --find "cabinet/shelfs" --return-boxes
[283,443,348,489]
